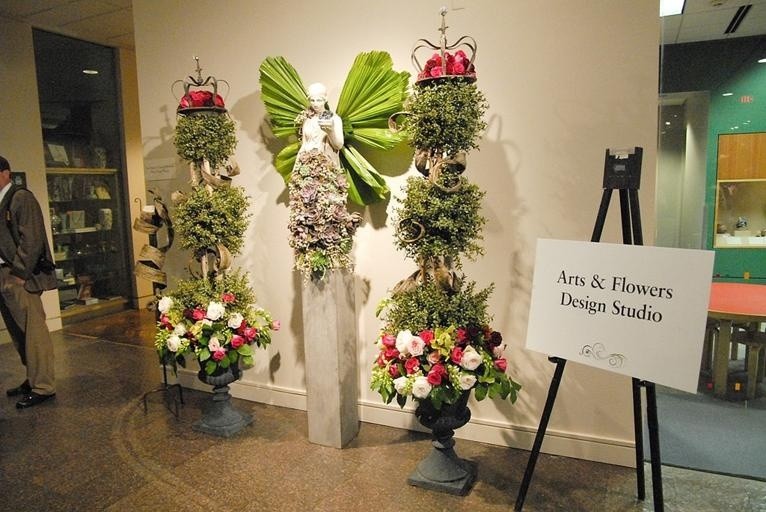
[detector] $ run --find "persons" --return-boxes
[294,82,345,171]
[0,157,60,408]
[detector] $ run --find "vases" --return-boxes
[405,387,480,498]
[191,352,257,440]
[299,267,360,450]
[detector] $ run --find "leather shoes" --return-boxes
[6,379,32,396]
[16,392,55,409]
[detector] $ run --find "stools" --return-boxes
[703,319,766,399]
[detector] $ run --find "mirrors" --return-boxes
[713,178,766,249]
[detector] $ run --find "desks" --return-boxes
[701,282,766,399]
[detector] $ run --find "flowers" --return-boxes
[367,50,522,410]
[285,152,363,288]
[153,90,281,376]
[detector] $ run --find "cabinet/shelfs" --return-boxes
[45,166,132,318]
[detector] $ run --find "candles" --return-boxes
[142,204,156,211]
